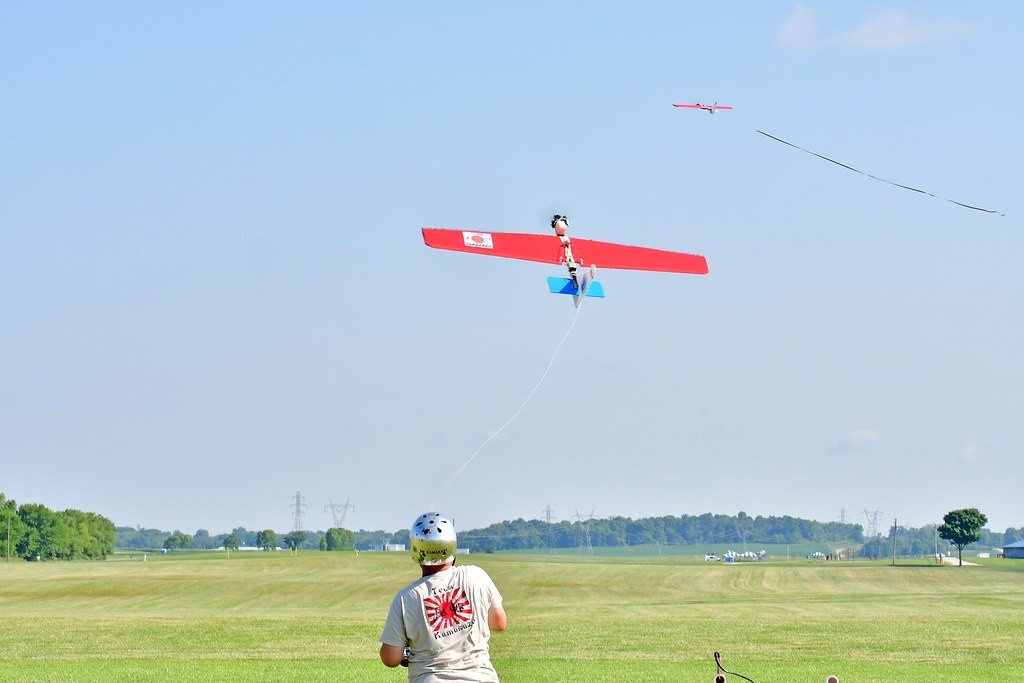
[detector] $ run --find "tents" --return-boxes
[813,552,826,561]
[723,550,759,562]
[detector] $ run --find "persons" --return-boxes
[380,512,506,683]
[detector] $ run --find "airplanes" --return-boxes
[420,213,709,309]
[672,100,735,114]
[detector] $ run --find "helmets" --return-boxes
[409,512,457,565]
